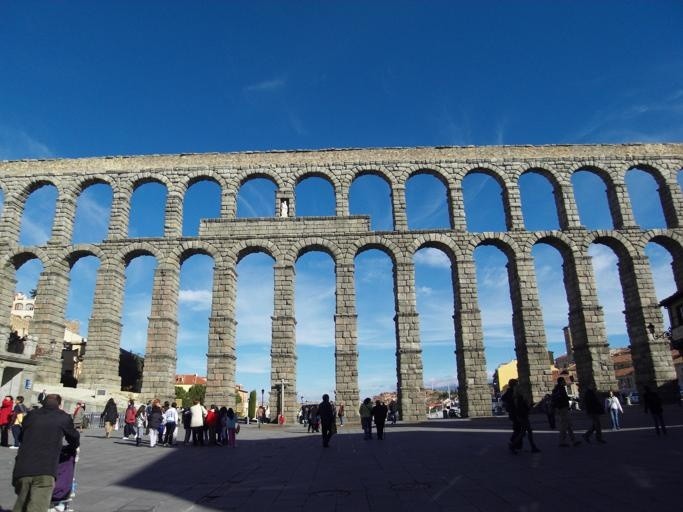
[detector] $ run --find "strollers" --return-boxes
[47,441,80,512]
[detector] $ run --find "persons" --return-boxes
[319,394,337,449]
[180,398,238,449]
[279,415,285,424]
[382,398,398,425]
[122,398,179,447]
[372,399,387,440]
[1,395,28,449]
[297,400,345,433]
[72,402,85,429]
[359,397,373,441]
[101,398,118,439]
[256,406,266,429]
[498,378,669,454]
[12,393,80,510]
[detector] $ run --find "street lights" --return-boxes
[304,400,308,404]
[300,396,303,405]
[261,389,263,406]
